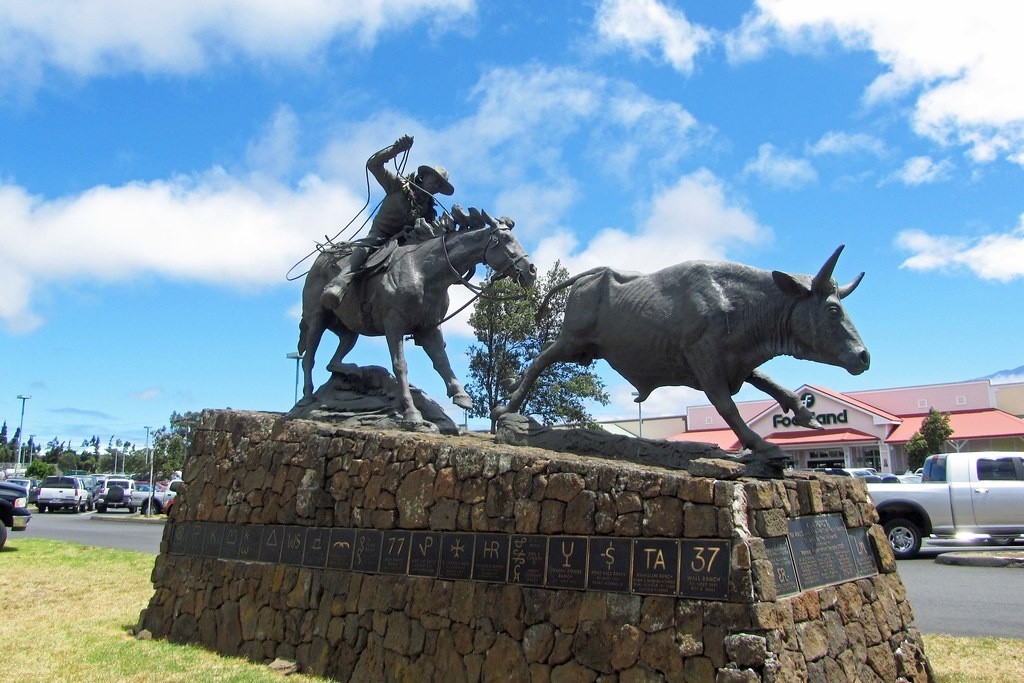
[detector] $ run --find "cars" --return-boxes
[802,465,924,483]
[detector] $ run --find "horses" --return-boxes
[297,208,538,426]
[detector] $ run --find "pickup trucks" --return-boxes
[863,451,1024,560]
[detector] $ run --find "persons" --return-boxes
[322,134,455,309]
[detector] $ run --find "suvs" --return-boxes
[0,470,184,550]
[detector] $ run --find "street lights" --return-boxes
[28,433,37,465]
[286,351,305,405]
[632,391,644,439]
[16,393,33,464]
[144,425,154,463]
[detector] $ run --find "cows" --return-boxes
[490,244,871,458]
